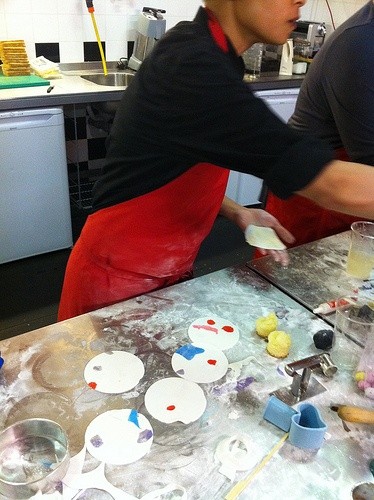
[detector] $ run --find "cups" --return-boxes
[346,221,374,279]
[330,294,374,370]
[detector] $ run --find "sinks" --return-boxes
[80,72,135,87]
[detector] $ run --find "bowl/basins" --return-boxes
[3,418,68,493]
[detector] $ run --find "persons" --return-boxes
[253,1,372,260]
[55,0,373,340]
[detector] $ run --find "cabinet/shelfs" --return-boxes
[221,87,301,208]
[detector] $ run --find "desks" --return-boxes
[0,231,374,498]
